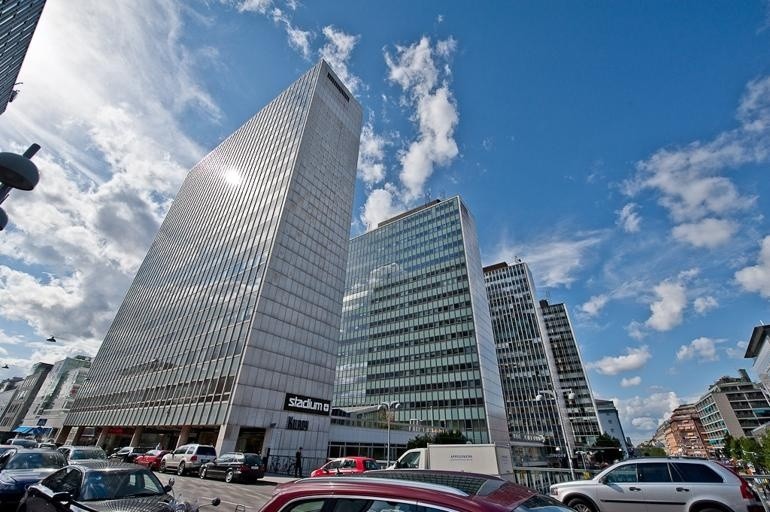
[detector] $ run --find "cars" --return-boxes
[549,456,758,511]
[258,470,580,512]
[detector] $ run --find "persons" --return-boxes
[156,442,162,451]
[295,447,304,478]
[551,457,608,483]
[75,438,106,451]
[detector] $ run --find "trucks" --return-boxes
[362,442,518,484]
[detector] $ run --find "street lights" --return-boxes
[536,388,578,480]
[376,400,400,467]
[2,364,32,369]
[46,334,99,349]
